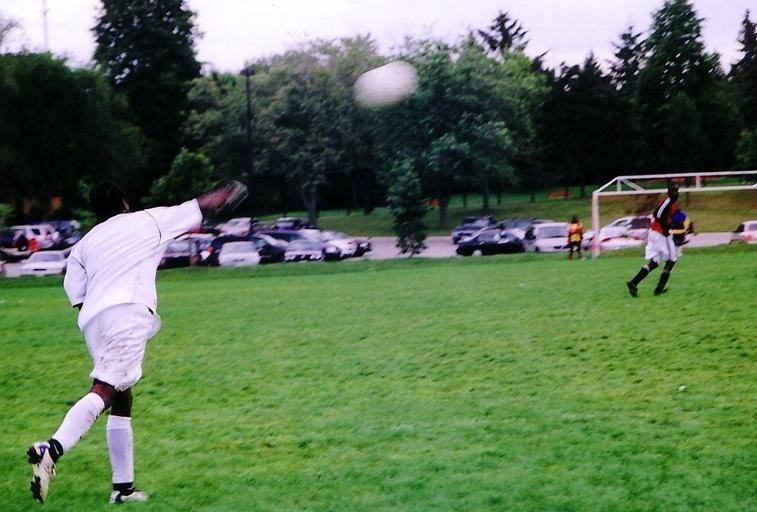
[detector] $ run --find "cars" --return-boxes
[0,215,370,280]
[449,214,673,257]
[729,220,757,245]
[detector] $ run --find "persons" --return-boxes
[24,180,252,506]
[626,183,692,297]
[565,213,587,257]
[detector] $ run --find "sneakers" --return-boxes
[628,283,638,296]
[26,442,56,504]
[109,487,146,504]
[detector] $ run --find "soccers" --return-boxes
[355,60,417,111]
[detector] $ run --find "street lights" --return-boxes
[238,61,261,228]
